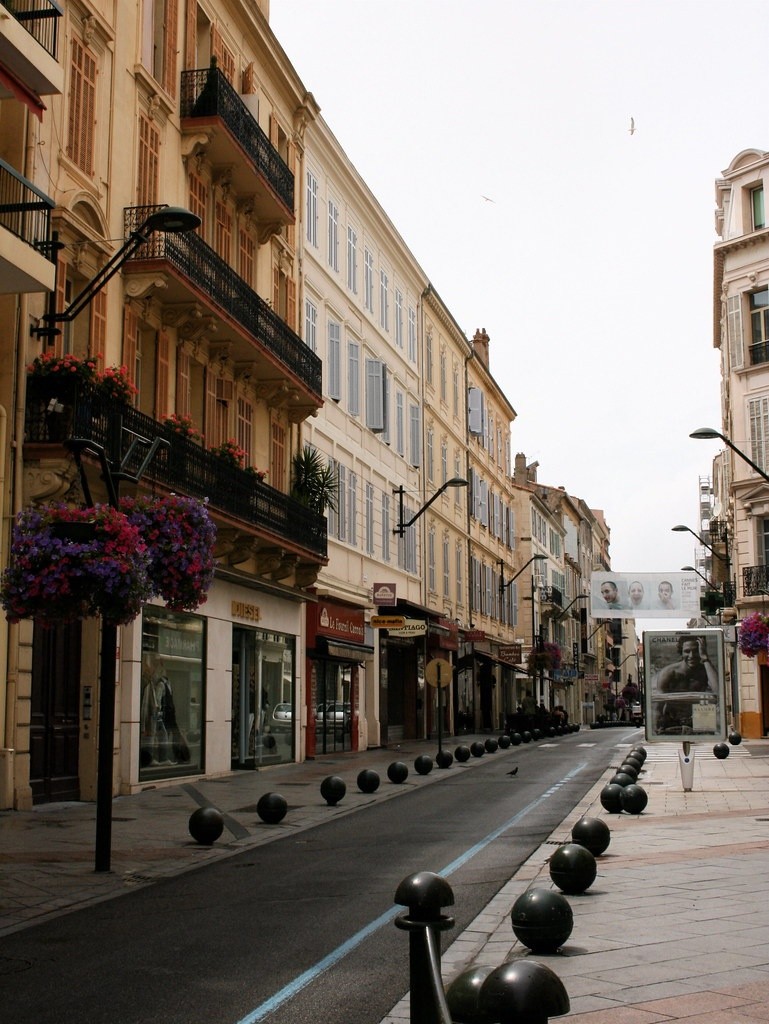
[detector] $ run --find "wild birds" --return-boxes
[505,765,520,778]
[628,117,637,135]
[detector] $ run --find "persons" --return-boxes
[650,636,718,735]
[600,581,620,604]
[651,581,675,610]
[140,665,178,766]
[628,581,645,605]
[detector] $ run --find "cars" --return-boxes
[325,703,359,729]
[315,703,329,732]
[268,703,292,732]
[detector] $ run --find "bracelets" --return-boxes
[700,659,709,663]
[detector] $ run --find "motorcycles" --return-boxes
[633,706,644,728]
